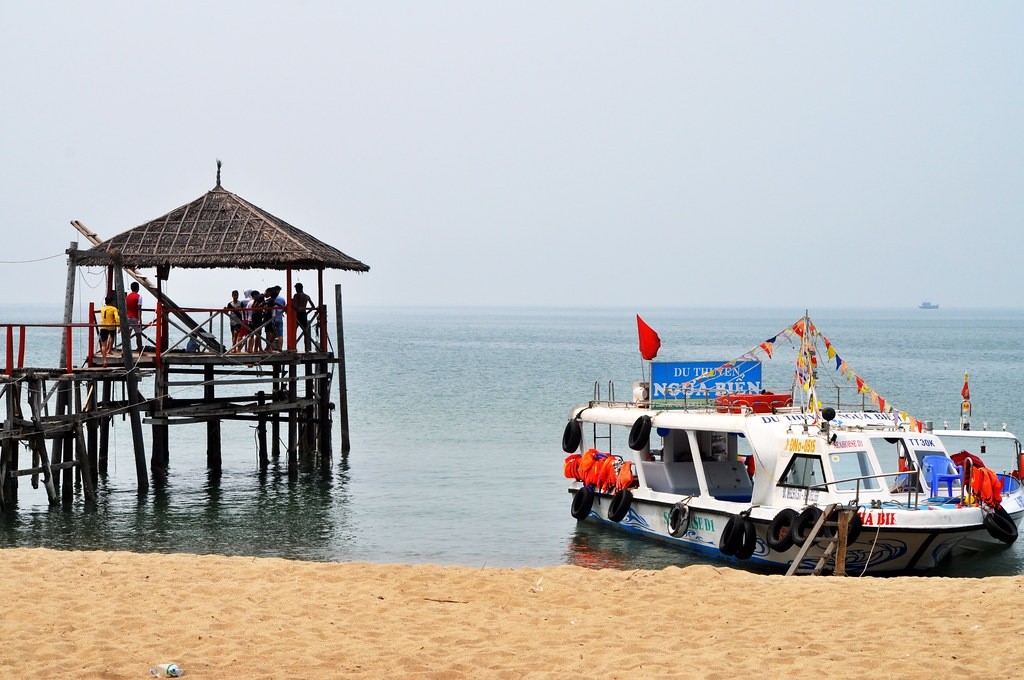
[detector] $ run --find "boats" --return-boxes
[890,371,1023,556]
[561,308,995,578]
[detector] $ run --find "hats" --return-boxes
[275,286,281,290]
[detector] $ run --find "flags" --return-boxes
[637,314,661,360]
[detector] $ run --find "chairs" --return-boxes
[923,456,963,498]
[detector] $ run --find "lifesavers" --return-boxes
[791,506,826,548]
[628,415,651,451]
[766,508,800,553]
[571,487,594,520]
[561,420,582,453]
[718,514,745,556]
[666,501,690,538]
[984,513,1018,541]
[735,518,756,560]
[607,488,633,522]
[824,508,862,547]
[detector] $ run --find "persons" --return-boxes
[224,283,315,354]
[126,282,148,356]
[187,335,200,352]
[99,295,119,368]
